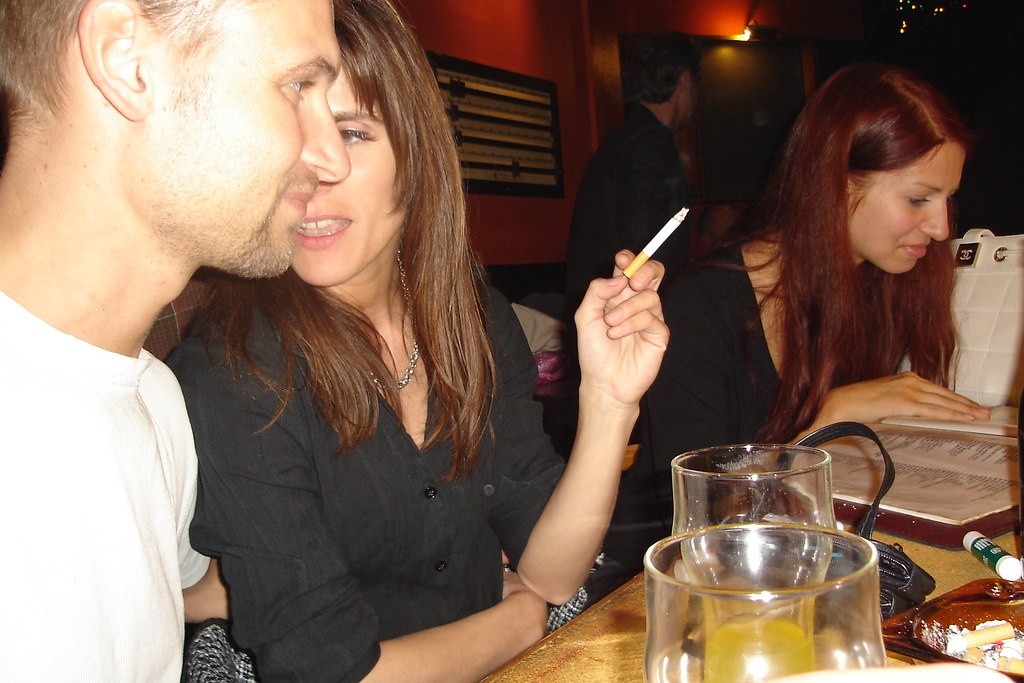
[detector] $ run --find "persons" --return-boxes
[644,60,989,536]
[0,0,351,683]
[559,32,702,323]
[160,0,671,683]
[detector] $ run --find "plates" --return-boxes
[882,578,1024,683]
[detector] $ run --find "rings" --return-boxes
[503,564,514,572]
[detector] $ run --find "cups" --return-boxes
[670,444,837,534]
[643,524,887,683]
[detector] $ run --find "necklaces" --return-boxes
[370,251,419,391]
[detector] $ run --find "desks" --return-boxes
[473,519,1023,683]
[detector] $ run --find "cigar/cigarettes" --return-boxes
[623,207,690,278]
[947,622,1024,675]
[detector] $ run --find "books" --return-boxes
[786,401,1024,550]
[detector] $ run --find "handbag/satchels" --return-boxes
[717,421,936,628]
[895,228,1024,406]
